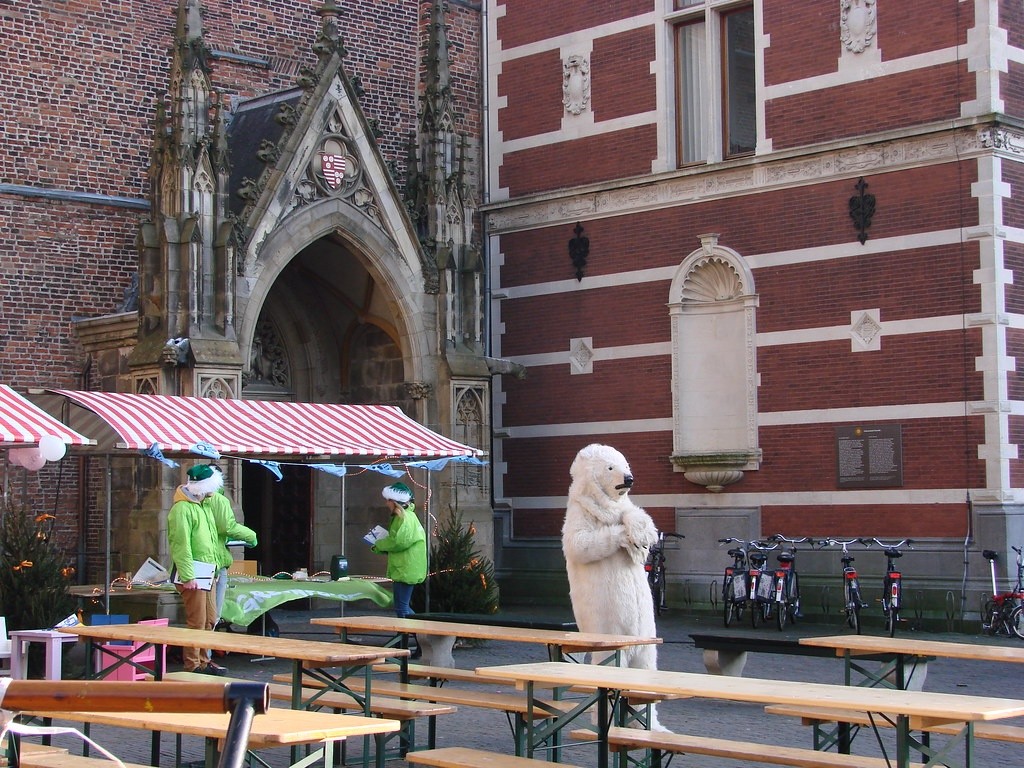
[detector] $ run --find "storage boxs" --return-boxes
[224,560,258,576]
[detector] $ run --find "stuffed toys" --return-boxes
[562,442,677,750]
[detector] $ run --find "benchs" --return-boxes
[0,616,1024,768]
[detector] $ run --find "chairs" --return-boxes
[0,616,31,680]
[101,618,169,681]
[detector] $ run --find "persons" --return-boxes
[370,481,428,661]
[166,464,258,676]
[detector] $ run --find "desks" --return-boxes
[474,662,1024,768]
[57,625,412,767]
[799,635,1024,761]
[310,613,663,763]
[8,630,80,681]
[19,707,401,768]
[47,575,395,662]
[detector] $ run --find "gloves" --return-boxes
[371,545,383,554]
[245,536,259,548]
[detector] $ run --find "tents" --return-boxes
[0,384,491,666]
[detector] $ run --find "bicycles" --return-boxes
[864,536,915,639]
[767,533,815,632]
[979,545,1024,641]
[644,531,686,620]
[746,538,785,628]
[716,537,769,628]
[817,536,872,635]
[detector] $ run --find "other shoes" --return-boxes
[193,661,228,677]
[391,645,423,658]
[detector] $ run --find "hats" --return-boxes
[184,464,224,495]
[382,482,414,502]
